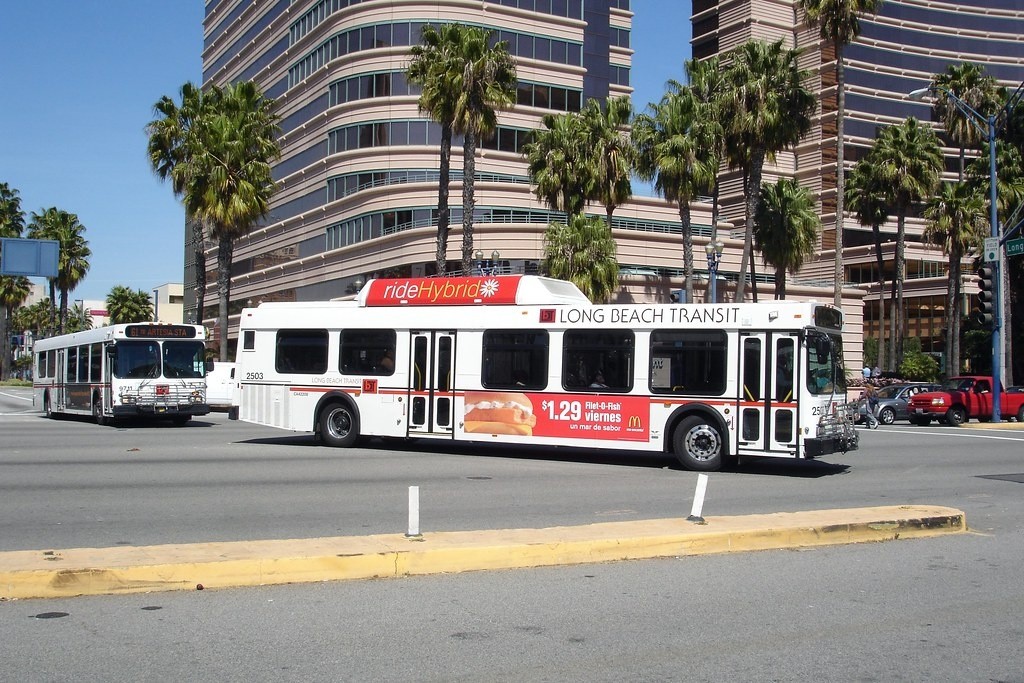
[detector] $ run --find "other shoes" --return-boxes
[874,421,880,428]
[865,425,870,429]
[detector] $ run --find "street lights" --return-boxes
[908,80,1024,420]
[152,289,159,322]
[705,240,724,303]
[74,299,84,331]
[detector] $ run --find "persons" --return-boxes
[379,348,393,372]
[763,354,793,400]
[861,364,870,379]
[588,371,610,389]
[858,383,881,429]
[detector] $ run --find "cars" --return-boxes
[852,384,942,425]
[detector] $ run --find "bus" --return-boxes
[229,273,860,472]
[32,321,215,426]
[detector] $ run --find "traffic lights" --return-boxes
[977,264,998,331]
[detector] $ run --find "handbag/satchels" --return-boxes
[869,396,879,404]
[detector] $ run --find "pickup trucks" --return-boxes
[906,376,1024,428]
